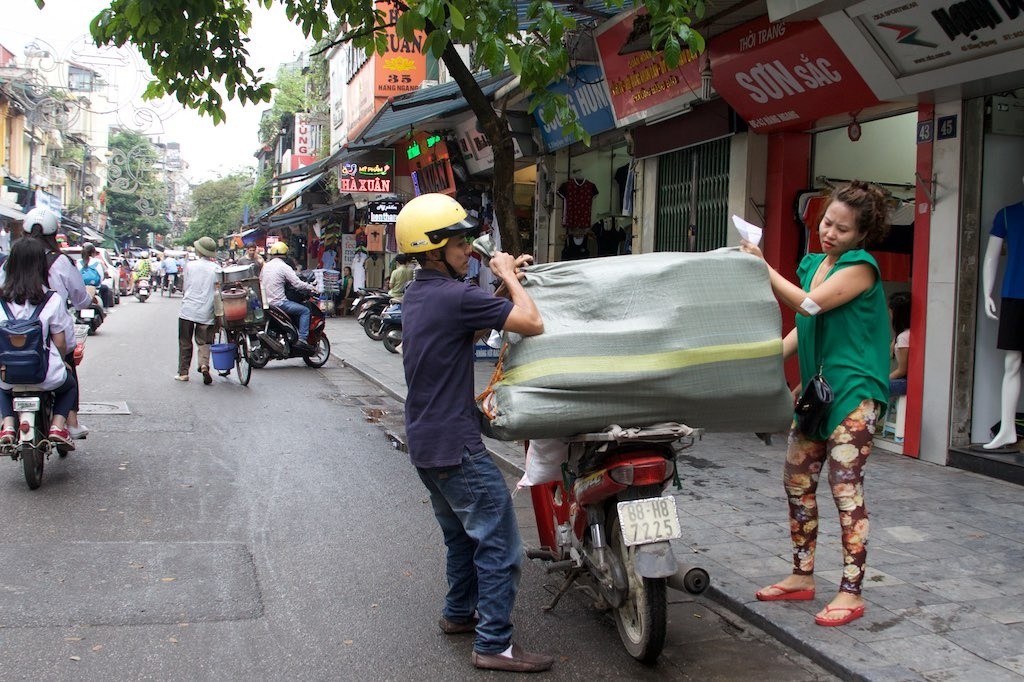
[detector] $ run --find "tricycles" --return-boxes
[161,273,186,298]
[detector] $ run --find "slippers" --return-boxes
[756,584,816,601]
[815,603,864,626]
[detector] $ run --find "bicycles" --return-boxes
[213,323,257,386]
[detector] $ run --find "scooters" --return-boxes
[75,285,108,335]
[152,273,159,292]
[136,277,152,302]
[7,324,91,486]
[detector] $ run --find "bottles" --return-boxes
[249,288,265,318]
[244,296,253,322]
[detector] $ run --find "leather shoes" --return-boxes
[439,612,481,634]
[472,637,555,672]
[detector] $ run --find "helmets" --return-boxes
[23,208,58,236]
[140,251,149,259]
[395,193,479,253]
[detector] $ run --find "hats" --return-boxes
[271,241,288,255]
[194,237,216,258]
[82,242,93,248]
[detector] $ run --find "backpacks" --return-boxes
[79,260,101,286]
[0,289,58,384]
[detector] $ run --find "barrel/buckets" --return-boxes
[211,342,236,370]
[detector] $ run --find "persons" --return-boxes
[881,290,911,398]
[1,213,430,310]
[174,237,226,384]
[0,239,79,453]
[1,208,99,439]
[391,193,562,673]
[742,181,892,626]
[258,240,318,348]
[981,200,1024,448]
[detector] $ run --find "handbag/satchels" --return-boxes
[794,373,833,438]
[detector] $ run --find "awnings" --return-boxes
[238,63,522,239]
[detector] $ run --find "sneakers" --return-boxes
[202,367,212,385]
[296,340,314,355]
[48,425,75,451]
[0,426,17,448]
[175,374,188,381]
[67,423,89,440]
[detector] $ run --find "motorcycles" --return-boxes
[244,271,333,369]
[351,286,404,353]
[514,422,711,663]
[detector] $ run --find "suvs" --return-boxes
[60,246,122,306]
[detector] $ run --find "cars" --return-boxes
[110,257,133,296]
